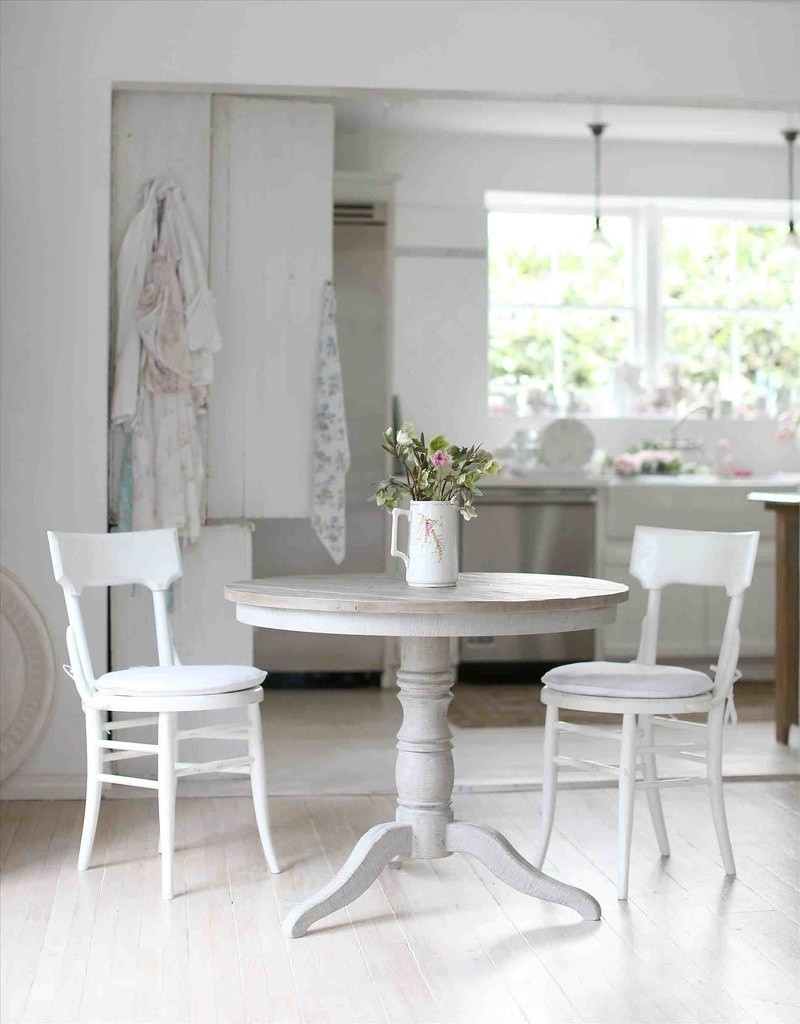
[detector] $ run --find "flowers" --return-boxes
[366,419,501,523]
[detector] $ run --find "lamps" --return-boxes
[578,121,614,259]
[777,131,800,260]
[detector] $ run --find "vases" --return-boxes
[389,501,460,588]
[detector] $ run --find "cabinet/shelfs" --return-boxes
[594,477,800,683]
[113,506,384,783]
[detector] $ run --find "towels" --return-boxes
[311,276,351,561]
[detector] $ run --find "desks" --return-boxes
[743,487,800,746]
[223,572,630,939]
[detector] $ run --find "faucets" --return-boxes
[671,402,713,445]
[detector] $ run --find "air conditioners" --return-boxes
[335,171,390,228]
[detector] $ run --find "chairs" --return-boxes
[538,524,761,901]
[46,525,281,897]
